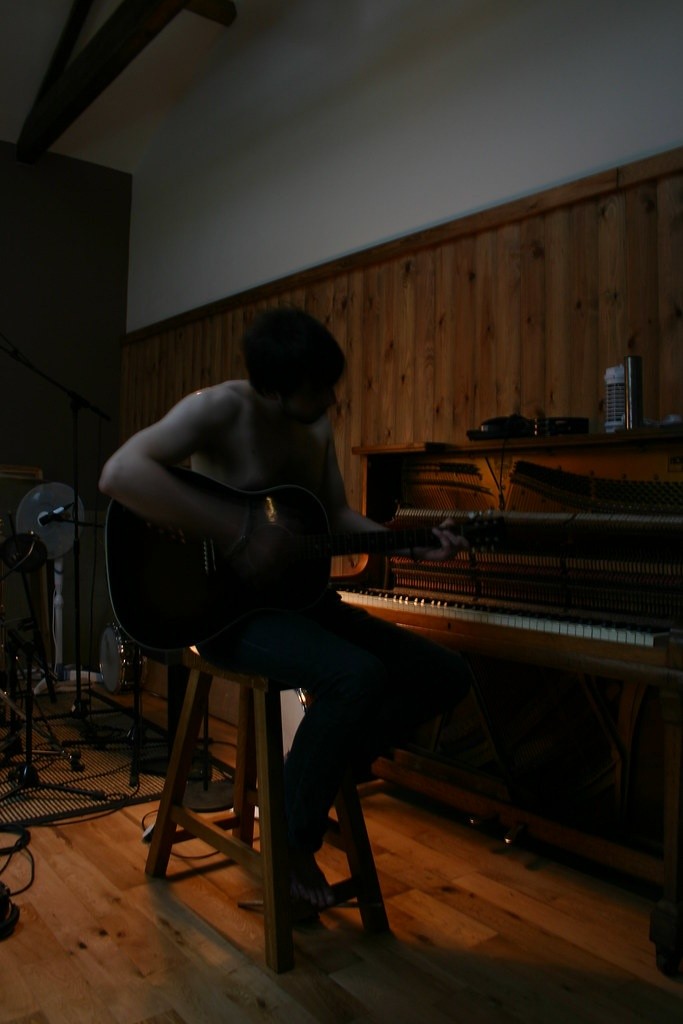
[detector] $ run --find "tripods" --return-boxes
[0,345,170,801]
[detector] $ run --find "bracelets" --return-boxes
[410,543,425,564]
[222,512,251,553]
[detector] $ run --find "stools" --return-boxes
[144,652,390,968]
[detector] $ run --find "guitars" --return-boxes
[104,469,495,658]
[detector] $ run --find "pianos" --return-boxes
[334,416,683,856]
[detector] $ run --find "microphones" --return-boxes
[38,501,76,526]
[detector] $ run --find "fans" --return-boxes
[16,484,101,694]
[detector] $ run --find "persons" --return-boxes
[98,309,472,905]
